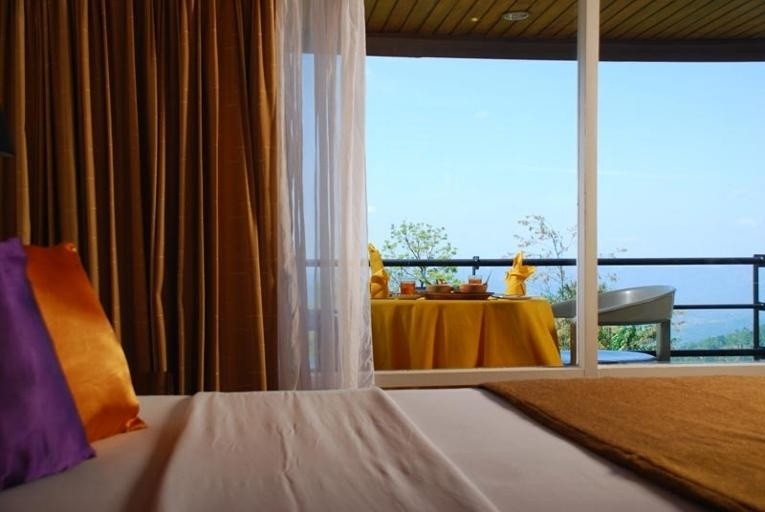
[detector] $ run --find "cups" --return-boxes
[468,275,482,285]
[400,280,416,296]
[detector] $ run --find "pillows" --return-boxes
[0,237,97,491]
[23,240,150,444]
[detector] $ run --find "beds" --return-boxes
[1,242,765,512]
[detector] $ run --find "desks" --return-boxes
[371,295,563,371]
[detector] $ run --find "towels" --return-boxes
[505,251,535,295]
[367,244,389,299]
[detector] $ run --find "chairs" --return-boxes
[552,285,677,366]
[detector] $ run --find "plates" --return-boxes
[398,294,420,301]
[505,294,532,301]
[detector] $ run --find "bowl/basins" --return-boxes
[460,284,488,294]
[425,284,451,293]
